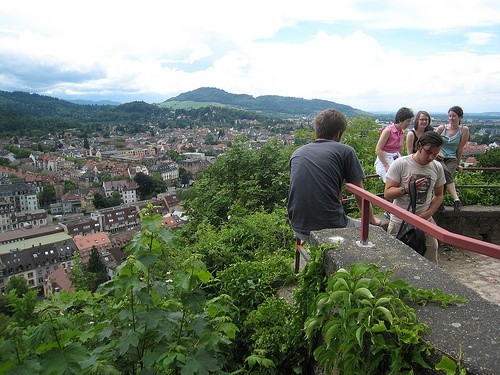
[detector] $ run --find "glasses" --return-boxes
[418,119,429,121]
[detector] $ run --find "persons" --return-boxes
[287,108,382,244]
[384,131,446,267]
[435,106,469,214]
[374,106,415,183]
[406,110,435,156]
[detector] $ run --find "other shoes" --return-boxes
[454,200,461,219]
[437,204,445,212]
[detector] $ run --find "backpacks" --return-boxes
[396,180,427,257]
